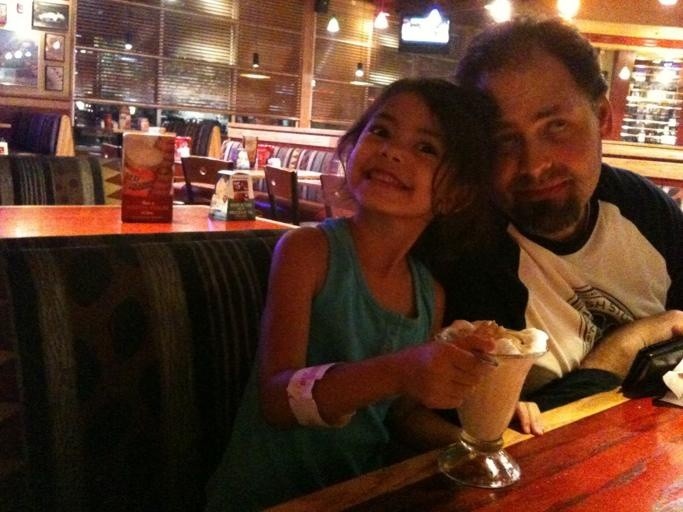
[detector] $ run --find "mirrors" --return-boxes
[0,26,41,89]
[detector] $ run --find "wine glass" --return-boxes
[430,327,548,490]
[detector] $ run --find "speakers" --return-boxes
[315,0,328,12]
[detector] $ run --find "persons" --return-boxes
[413,19,683,414]
[213,72,544,511]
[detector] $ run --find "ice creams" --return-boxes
[434,318,550,441]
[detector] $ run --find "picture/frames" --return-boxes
[42,32,66,63]
[30,0,70,32]
[44,65,63,92]
[0,3,8,24]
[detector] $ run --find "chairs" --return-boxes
[74,104,360,227]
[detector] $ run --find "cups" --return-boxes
[103,112,113,131]
[269,158,281,168]
[243,136,257,168]
[0,142,7,155]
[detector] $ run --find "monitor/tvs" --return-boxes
[398,12,452,54]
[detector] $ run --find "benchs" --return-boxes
[0,155,104,207]
[10,110,73,157]
[0,228,289,511]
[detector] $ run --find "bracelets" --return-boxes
[285,361,358,429]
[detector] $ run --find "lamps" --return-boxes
[326,1,340,36]
[250,0,261,69]
[374,1,389,31]
[123,0,132,51]
[354,0,364,77]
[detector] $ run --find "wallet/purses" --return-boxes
[620,334,682,394]
